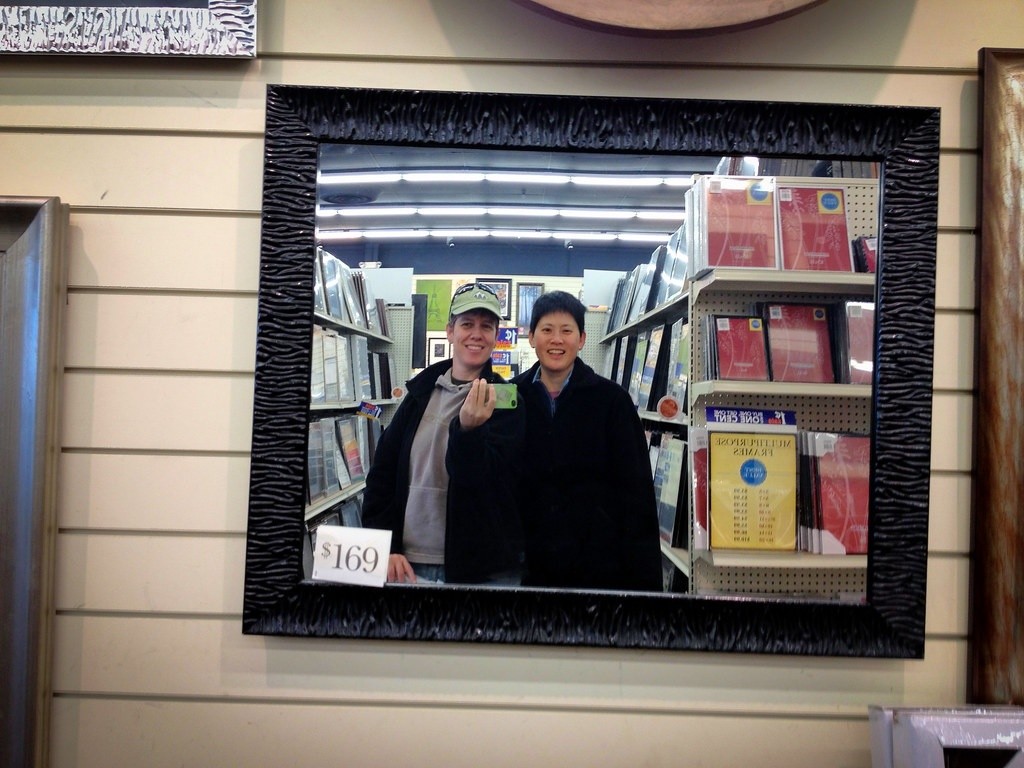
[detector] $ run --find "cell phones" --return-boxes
[485,384,517,408]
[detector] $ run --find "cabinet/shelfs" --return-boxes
[300,158,881,601]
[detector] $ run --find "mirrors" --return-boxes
[241,81,941,660]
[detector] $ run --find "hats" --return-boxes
[450,283,503,320]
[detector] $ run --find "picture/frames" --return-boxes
[0,0,257,60]
[0,196,71,768]
[428,338,450,364]
[515,282,545,338]
[476,278,512,320]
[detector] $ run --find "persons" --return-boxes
[362,284,664,593]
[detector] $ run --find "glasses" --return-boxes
[449,282,498,321]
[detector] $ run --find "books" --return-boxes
[604,225,687,594]
[687,158,881,282]
[302,251,397,581]
[693,430,872,555]
[705,298,875,385]
